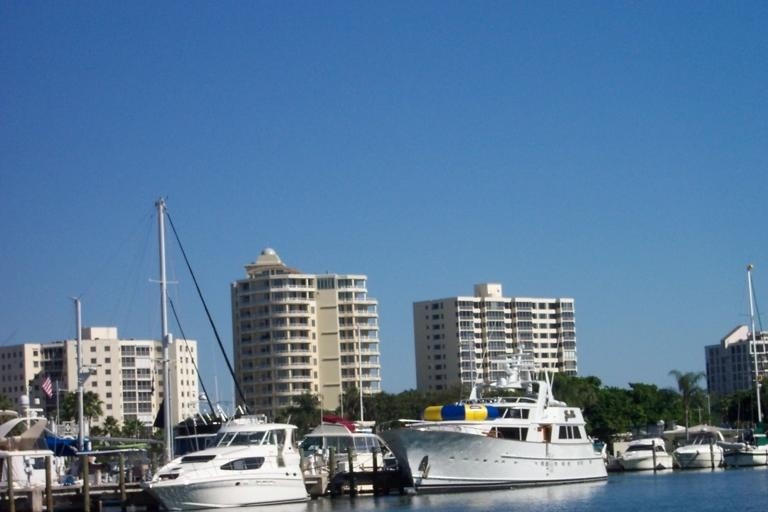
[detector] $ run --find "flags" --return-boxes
[41,373,54,399]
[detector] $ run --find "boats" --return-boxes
[597,430,768,470]
[378,379,607,495]
[142,411,399,510]
[0,449,83,486]
